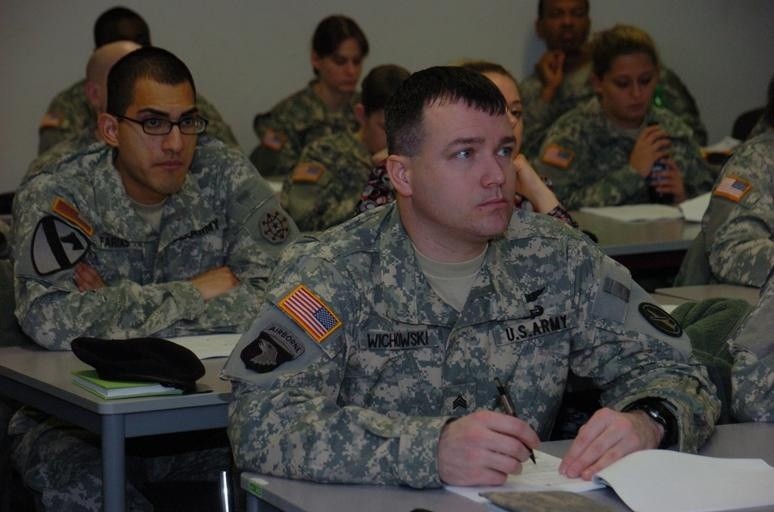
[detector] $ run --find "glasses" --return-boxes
[119,115,209,136]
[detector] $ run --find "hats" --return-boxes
[69,334,207,392]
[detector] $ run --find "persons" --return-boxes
[666,76,774,290]
[215,60,729,493]
[249,15,579,231]
[519,0,715,210]
[25,5,237,182]
[2,43,306,511]
[717,259,773,430]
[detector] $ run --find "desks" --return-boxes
[2,281,774,512]
[569,205,702,259]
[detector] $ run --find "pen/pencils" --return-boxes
[494,377,536,465]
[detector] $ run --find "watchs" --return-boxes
[641,401,676,448]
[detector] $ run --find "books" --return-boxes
[70,369,184,402]
[444,444,774,512]
